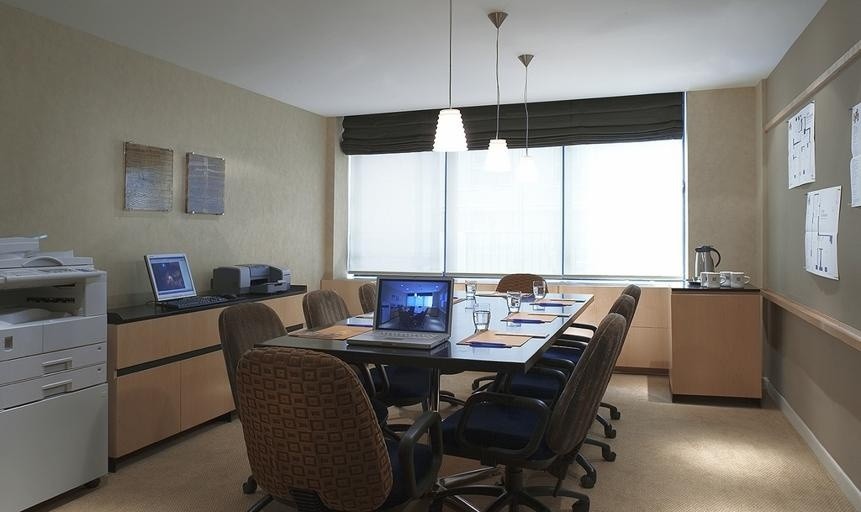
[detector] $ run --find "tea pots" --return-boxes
[695,246,721,277]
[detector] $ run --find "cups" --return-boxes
[701,271,716,287]
[731,272,751,288]
[533,298,545,311]
[465,298,479,312]
[532,282,548,301]
[506,313,522,328]
[463,279,478,299]
[474,340,490,356]
[473,305,492,334]
[708,273,727,288]
[506,293,522,313]
[719,272,732,287]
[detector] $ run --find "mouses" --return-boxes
[221,293,238,299]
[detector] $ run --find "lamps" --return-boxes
[431,0,468,152]
[484,9,512,166]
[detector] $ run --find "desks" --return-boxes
[253,289,595,474]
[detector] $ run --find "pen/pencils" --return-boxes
[513,320,546,324]
[540,303,563,306]
[469,343,513,348]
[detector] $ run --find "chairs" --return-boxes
[234,346,445,512]
[471,276,550,391]
[301,282,376,330]
[218,303,289,421]
[435,284,640,512]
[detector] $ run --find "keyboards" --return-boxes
[168,296,229,310]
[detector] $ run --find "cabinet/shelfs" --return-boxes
[106,284,308,469]
[668,280,763,406]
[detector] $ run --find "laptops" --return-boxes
[347,275,454,349]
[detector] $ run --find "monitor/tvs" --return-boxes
[144,253,197,302]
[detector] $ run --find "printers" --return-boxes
[213,263,292,296]
